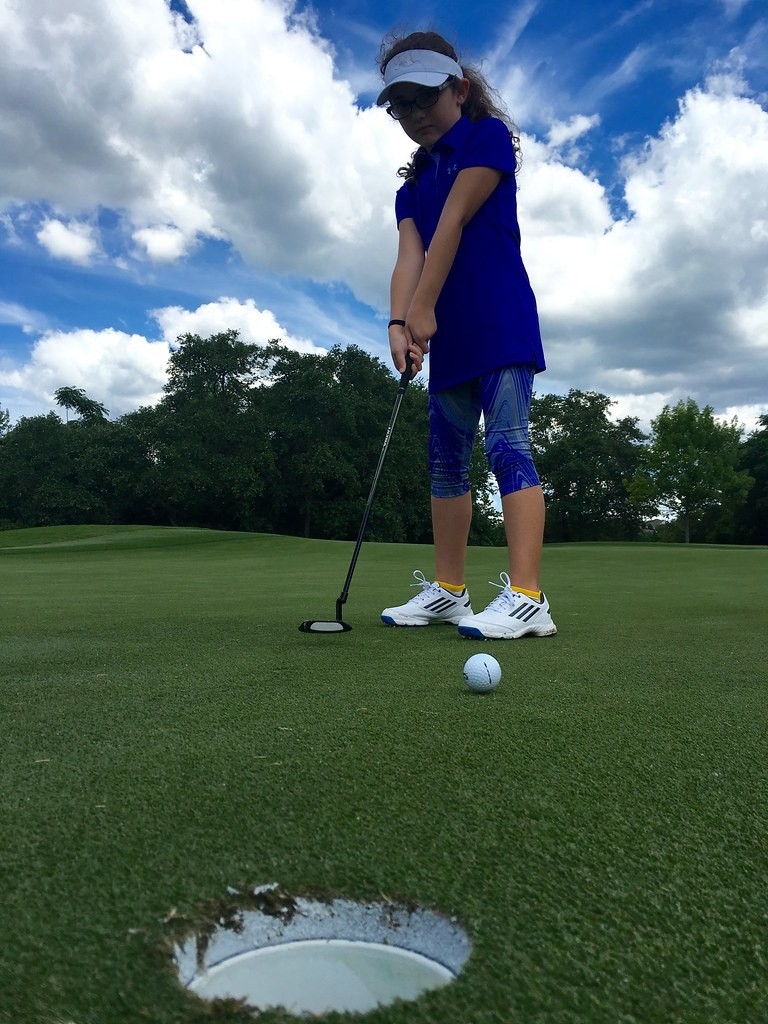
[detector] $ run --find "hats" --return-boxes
[376,48,463,106]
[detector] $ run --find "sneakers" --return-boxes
[380,570,475,627]
[457,572,558,642]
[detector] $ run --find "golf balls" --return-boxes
[461,650,505,692]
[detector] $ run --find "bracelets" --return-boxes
[388,319,406,329]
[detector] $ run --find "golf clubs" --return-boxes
[299,355,412,632]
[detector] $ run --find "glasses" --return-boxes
[386,78,456,120]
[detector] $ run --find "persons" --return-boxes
[376,31,558,639]
